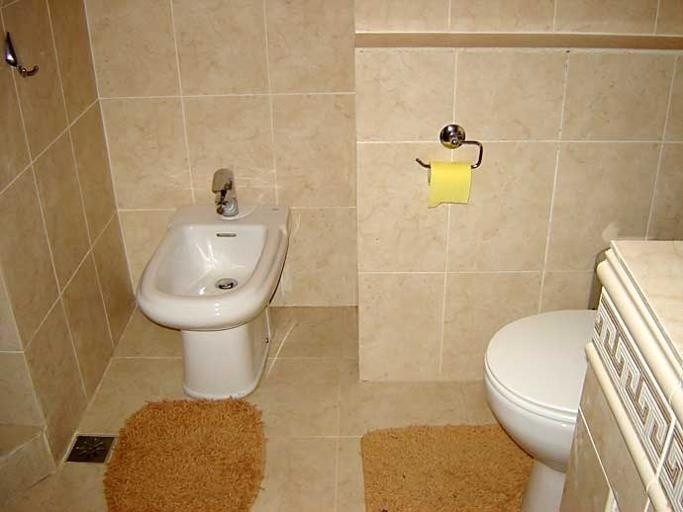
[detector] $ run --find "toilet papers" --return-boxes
[429,161,473,208]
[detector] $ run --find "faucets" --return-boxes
[210,168,240,216]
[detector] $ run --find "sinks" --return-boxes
[135,203,294,331]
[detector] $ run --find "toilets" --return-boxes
[482,309,598,512]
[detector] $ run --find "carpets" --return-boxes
[360,420,532,512]
[104,399,265,512]
